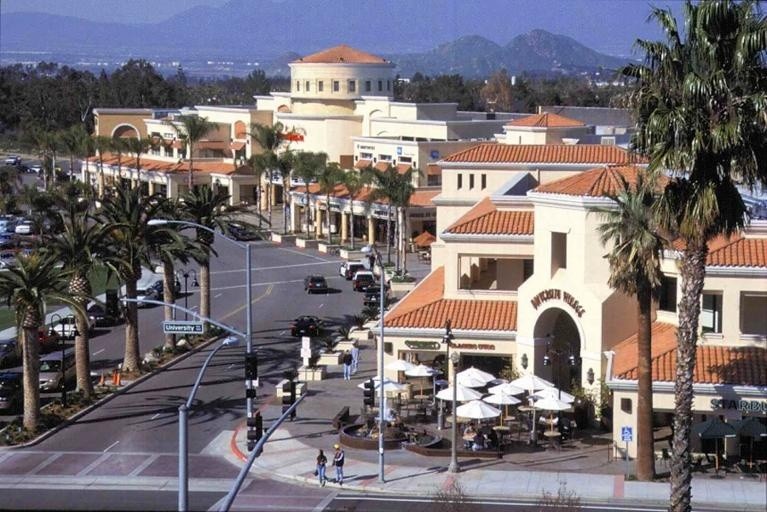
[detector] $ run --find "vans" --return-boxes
[37,351,76,390]
[0,326,22,366]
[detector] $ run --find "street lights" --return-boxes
[145,219,252,356]
[174,268,198,339]
[177,336,239,512]
[448,351,461,472]
[50,311,67,409]
[543,340,574,401]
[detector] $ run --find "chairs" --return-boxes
[660,448,767,480]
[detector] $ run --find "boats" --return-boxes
[279,384,295,418]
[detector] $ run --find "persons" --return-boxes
[368,251,376,269]
[315,448,328,488]
[342,349,354,382]
[331,443,345,485]
[350,342,360,374]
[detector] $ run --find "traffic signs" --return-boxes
[164,320,202,333]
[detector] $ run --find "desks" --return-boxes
[378,391,569,452]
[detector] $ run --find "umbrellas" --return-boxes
[693,414,736,471]
[731,414,766,469]
[356,356,577,449]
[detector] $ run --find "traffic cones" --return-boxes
[112,369,120,384]
[114,373,123,387]
[98,372,105,385]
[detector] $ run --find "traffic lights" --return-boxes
[246,357,256,379]
[363,378,374,408]
[245,416,262,455]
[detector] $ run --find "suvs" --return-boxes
[363,279,388,308]
[303,274,328,296]
[5,155,22,165]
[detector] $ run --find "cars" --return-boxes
[221,220,252,240]
[54,165,60,171]
[150,279,181,298]
[52,315,95,339]
[351,270,376,290]
[121,287,158,308]
[88,302,125,324]
[39,328,60,348]
[0,213,59,270]
[291,315,323,337]
[27,164,42,174]
[338,259,365,277]
[0,370,24,410]
